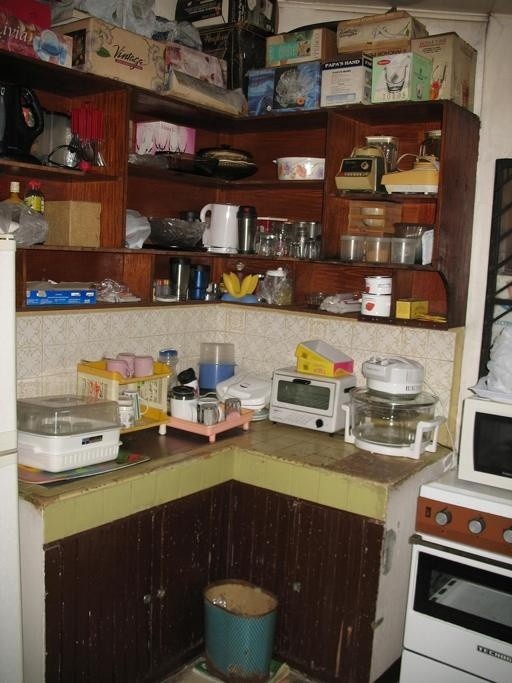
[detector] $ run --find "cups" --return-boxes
[123,391,149,422]
[117,353,136,377]
[199,404,218,426]
[224,398,242,421]
[106,360,131,379]
[117,395,135,430]
[134,355,153,378]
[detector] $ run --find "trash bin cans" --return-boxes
[202,579,280,683]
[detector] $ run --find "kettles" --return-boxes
[199,201,240,255]
[0,82,44,165]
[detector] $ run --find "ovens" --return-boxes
[402,541,512,683]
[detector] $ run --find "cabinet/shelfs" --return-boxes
[0,47,480,329]
[17,489,230,681]
[231,454,454,683]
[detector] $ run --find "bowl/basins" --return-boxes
[272,156,325,182]
[360,207,385,228]
[145,217,206,249]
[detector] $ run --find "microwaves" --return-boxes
[456,395,512,492]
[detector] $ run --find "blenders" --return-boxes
[342,354,446,459]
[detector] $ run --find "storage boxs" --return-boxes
[53,16,166,91]
[247,19,479,112]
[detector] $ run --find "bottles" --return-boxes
[1,181,25,206]
[23,179,46,245]
[169,386,198,423]
[159,347,178,391]
[236,205,257,254]
[391,237,418,265]
[170,255,211,303]
[339,235,365,262]
[365,236,391,264]
